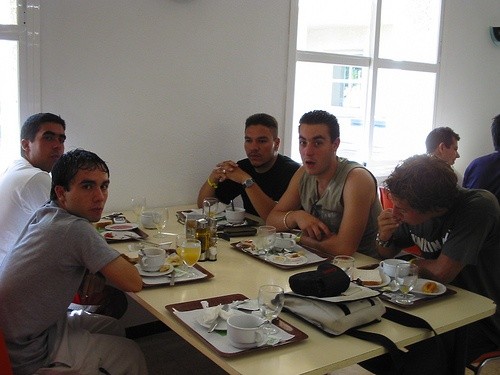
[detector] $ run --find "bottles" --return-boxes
[195,217,219,262]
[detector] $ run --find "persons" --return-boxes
[425,127,461,166]
[1,147,148,374]
[376,154,500,375]
[462,114,500,205]
[266,110,385,261]
[0,113,127,333]
[197,114,300,216]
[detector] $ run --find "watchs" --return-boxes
[242,178,256,189]
[296,233,304,242]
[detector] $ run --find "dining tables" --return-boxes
[89,203,497,375]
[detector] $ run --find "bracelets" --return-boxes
[207,178,218,189]
[376,232,392,248]
[284,210,297,231]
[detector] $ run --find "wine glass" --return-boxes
[181,238,199,278]
[204,197,218,219]
[392,262,419,305]
[257,225,276,261]
[175,233,194,272]
[152,206,170,239]
[258,284,285,334]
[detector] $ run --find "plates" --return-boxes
[411,278,446,296]
[100,223,139,240]
[133,263,174,276]
[227,339,265,349]
[269,252,307,267]
[199,309,227,331]
[356,270,390,288]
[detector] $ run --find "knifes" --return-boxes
[208,304,229,333]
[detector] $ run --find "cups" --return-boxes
[379,259,411,278]
[139,247,165,271]
[141,212,159,229]
[224,207,246,224]
[275,232,296,250]
[332,255,356,280]
[225,314,266,343]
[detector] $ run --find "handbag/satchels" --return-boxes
[272,281,443,374]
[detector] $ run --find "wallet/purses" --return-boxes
[288,263,350,296]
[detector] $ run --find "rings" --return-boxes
[222,169,225,173]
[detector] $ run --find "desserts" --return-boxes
[423,281,438,292]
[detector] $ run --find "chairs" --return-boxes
[380,186,420,254]
